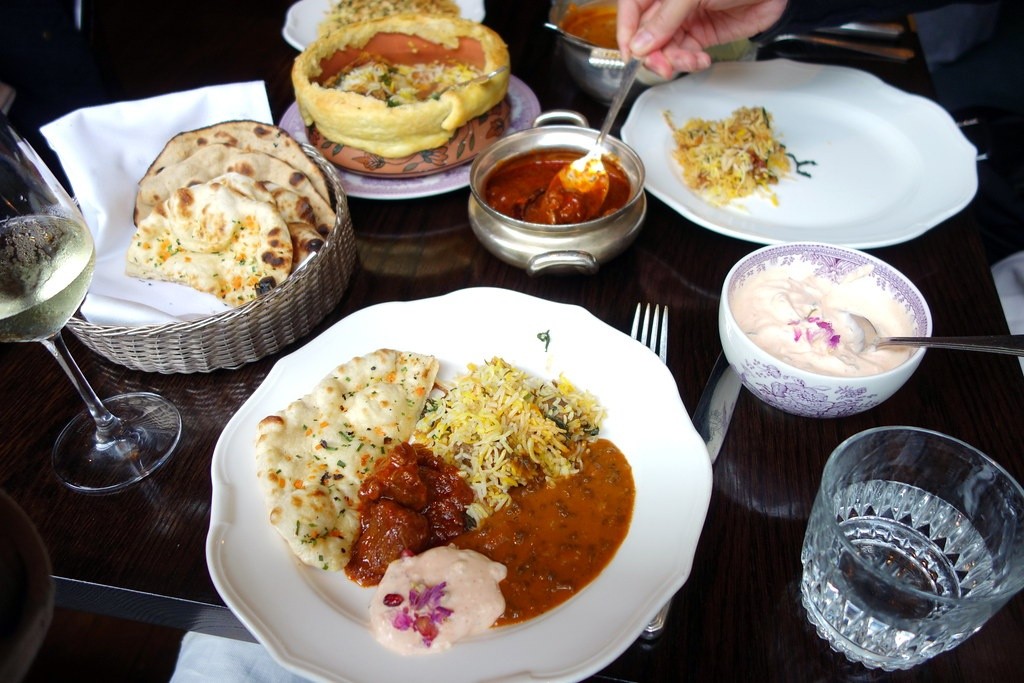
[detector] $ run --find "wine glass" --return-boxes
[0,112,184,495]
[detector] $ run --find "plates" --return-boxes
[283,0,486,53]
[205,286,714,683]
[279,75,542,200]
[621,58,978,250]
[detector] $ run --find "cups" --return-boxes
[800,425,1024,672]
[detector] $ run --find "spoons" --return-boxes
[543,53,644,219]
[849,313,1024,357]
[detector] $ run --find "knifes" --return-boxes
[642,350,742,640]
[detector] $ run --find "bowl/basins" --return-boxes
[469,110,647,277]
[0,488,56,683]
[719,242,934,418]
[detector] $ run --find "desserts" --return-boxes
[732,255,918,376]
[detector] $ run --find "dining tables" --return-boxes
[0,0,1024,683]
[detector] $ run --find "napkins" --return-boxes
[37,77,316,333]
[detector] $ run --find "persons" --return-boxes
[0,0,116,221]
[617,1,1024,253]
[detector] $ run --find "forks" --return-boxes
[630,303,668,365]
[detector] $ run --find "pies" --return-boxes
[255,349,440,571]
[127,121,336,310]
